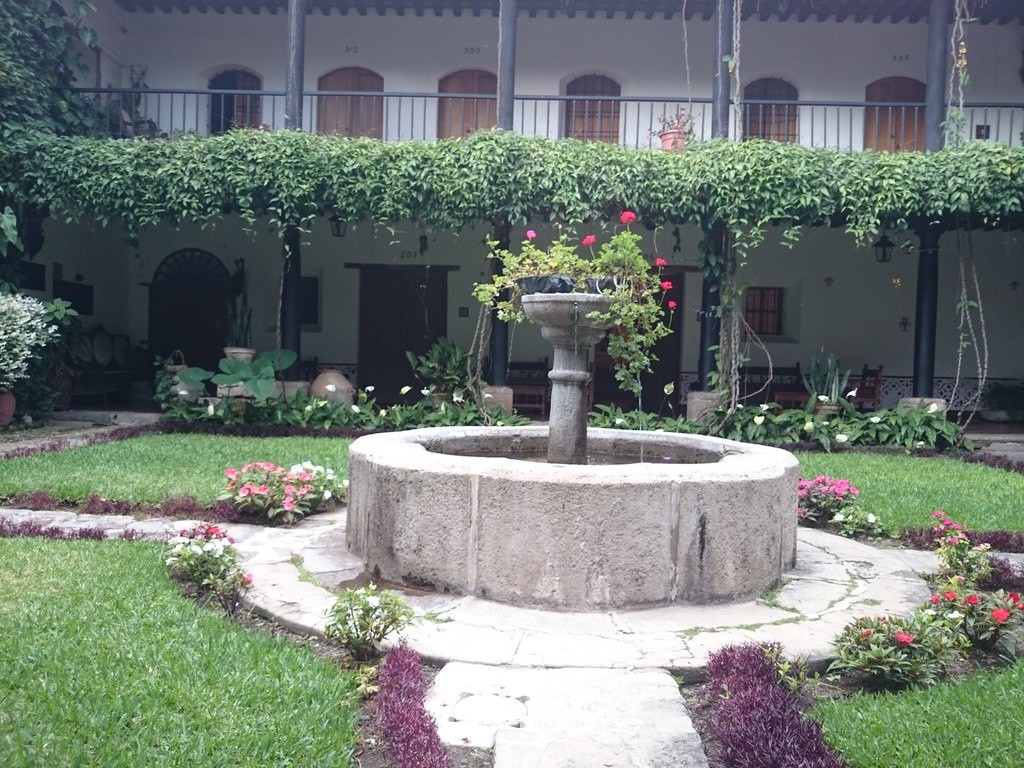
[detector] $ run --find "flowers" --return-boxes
[476,212,676,394]
[647,107,692,140]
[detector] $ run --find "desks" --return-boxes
[774,384,811,416]
[509,382,547,415]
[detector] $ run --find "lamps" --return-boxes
[871,231,895,262]
[329,216,347,237]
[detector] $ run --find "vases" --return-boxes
[514,275,618,295]
[658,129,686,152]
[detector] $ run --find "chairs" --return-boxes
[119,109,156,139]
[845,364,884,414]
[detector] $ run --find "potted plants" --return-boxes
[217,292,255,364]
[801,345,853,422]
[405,336,468,410]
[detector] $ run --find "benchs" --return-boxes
[69,324,136,408]
